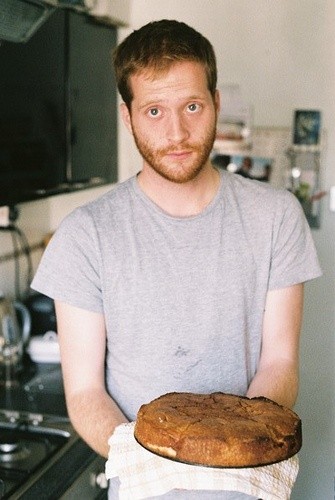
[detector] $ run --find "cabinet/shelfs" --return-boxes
[0,0,119,206]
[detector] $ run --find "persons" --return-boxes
[30,20,324,500]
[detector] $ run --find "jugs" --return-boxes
[0,292,31,370]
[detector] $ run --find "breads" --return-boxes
[133,391,303,468]
